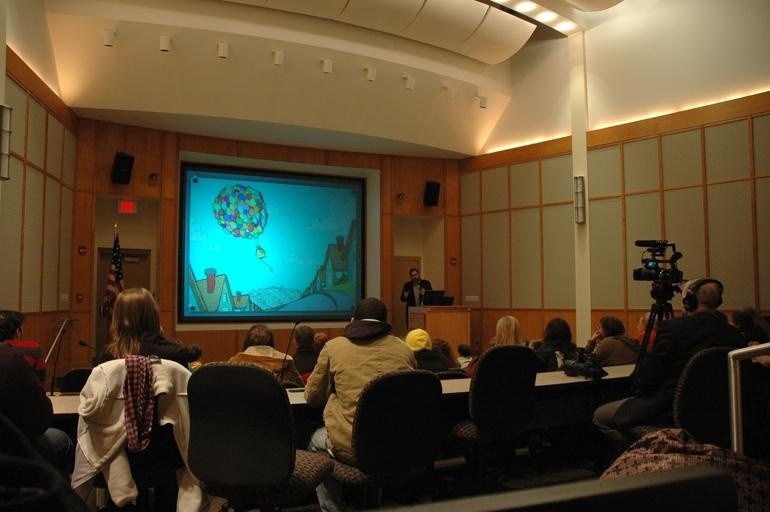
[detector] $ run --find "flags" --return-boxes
[98,231,128,344]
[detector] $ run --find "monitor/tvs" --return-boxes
[423,289,454,306]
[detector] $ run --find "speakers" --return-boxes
[422,180,440,208]
[111,151,135,185]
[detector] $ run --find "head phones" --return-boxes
[682,277,724,312]
[242,324,275,348]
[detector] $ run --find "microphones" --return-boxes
[78,341,104,353]
[280,319,302,389]
[49,329,67,396]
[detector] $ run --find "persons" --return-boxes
[301,297,420,512]
[105,285,189,368]
[314,333,330,348]
[292,325,322,374]
[227,322,305,388]
[731,307,770,345]
[492,312,666,371]
[406,329,496,378]
[399,267,432,328]
[591,277,754,474]
[0,339,76,488]
[0,307,50,389]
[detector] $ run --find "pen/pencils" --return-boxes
[289,390,305,392]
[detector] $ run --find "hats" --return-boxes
[406,328,433,352]
